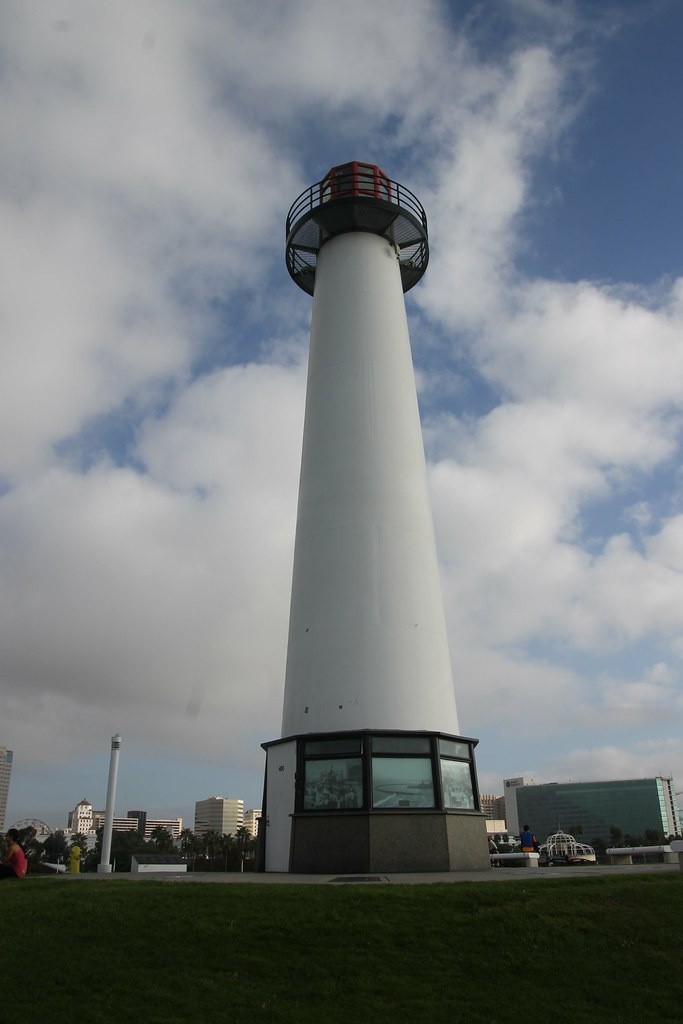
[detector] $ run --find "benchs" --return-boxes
[489,853,539,868]
[606,840,683,865]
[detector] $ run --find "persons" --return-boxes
[1,828,27,880]
[520,824,537,851]
